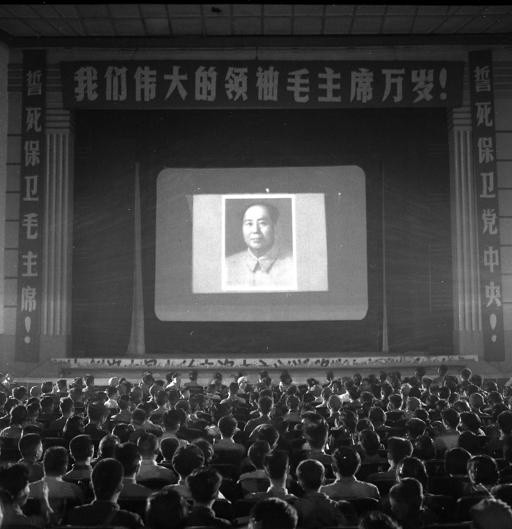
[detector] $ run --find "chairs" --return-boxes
[0,377,512,528]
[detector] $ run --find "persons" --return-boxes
[224,200,296,288]
[0,361,511,528]
